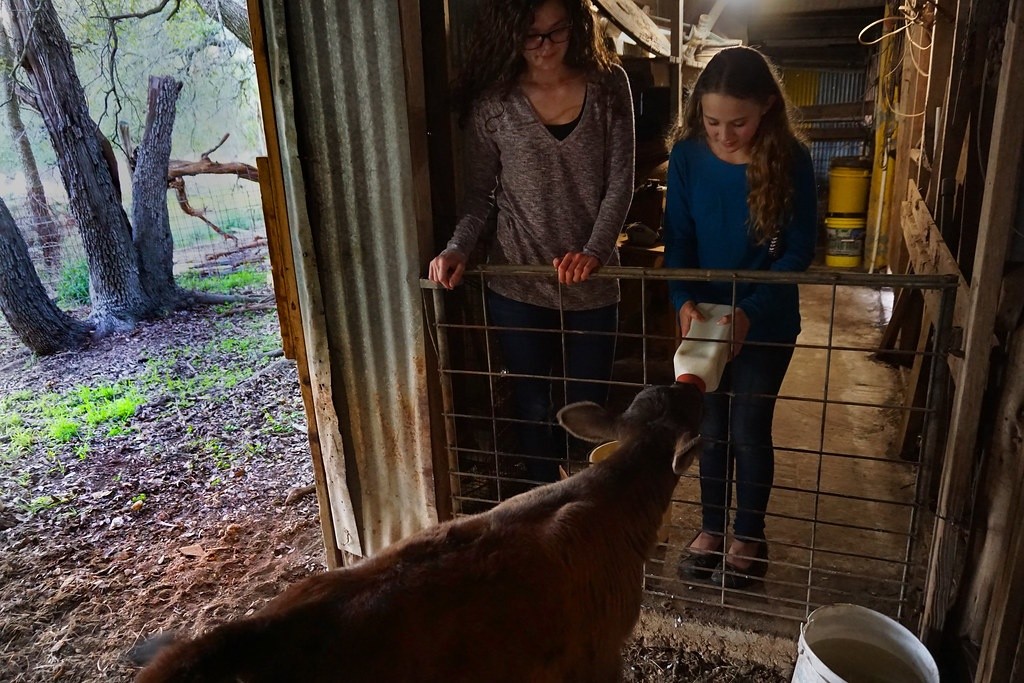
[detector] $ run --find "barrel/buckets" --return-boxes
[827,167,869,215]
[824,218,866,268]
[792,602,940,683]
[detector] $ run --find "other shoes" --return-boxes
[710,541,769,589]
[674,529,727,580]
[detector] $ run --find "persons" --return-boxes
[427,0,636,495]
[662,42,817,588]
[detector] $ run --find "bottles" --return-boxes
[673,305,735,393]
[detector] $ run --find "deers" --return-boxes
[131,382,708,683]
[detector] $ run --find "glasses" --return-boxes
[522,19,574,50]
